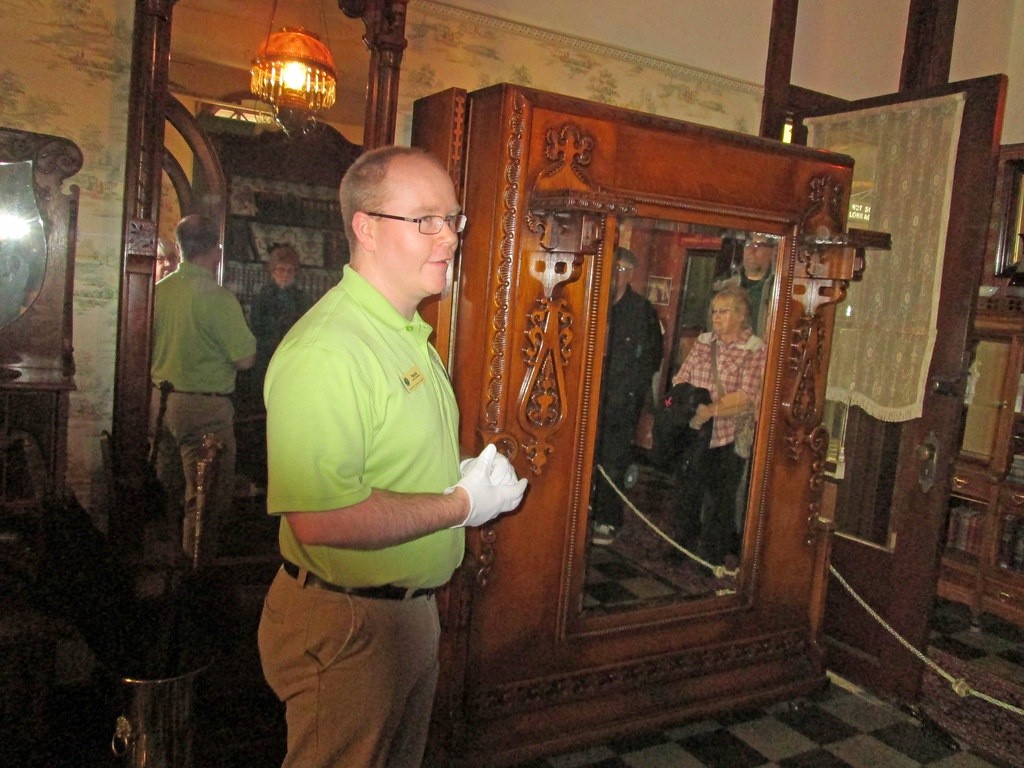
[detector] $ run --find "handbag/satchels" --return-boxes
[734,414,755,459]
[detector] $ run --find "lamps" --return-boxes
[238,2,339,133]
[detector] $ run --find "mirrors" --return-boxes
[580,222,799,618]
[110,0,405,765]
[0,143,84,388]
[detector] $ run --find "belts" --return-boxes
[151,381,220,396]
[284,559,435,598]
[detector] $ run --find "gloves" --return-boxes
[444,444,528,528]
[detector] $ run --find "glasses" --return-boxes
[272,267,296,274]
[365,213,468,235]
[616,265,632,272]
[744,241,776,249]
[712,308,736,313]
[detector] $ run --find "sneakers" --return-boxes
[592,525,622,545]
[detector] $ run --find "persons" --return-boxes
[593,248,663,545]
[143,214,257,570]
[255,148,527,768]
[255,245,321,370]
[664,231,776,594]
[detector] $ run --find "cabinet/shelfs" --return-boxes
[385,90,893,768]
[935,141,1024,619]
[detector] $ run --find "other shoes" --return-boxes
[698,556,725,574]
[662,546,698,566]
